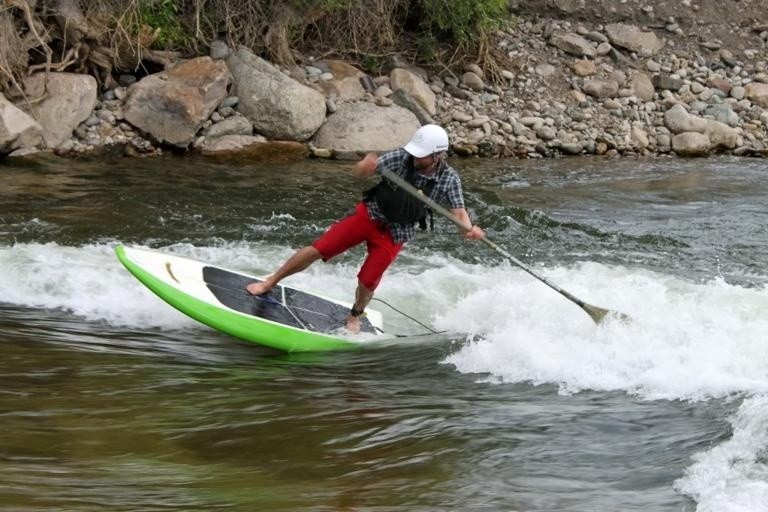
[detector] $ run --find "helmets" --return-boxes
[402,124,449,159]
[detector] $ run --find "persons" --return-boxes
[245,124,486,332]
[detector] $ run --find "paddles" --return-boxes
[375,165,637,331]
